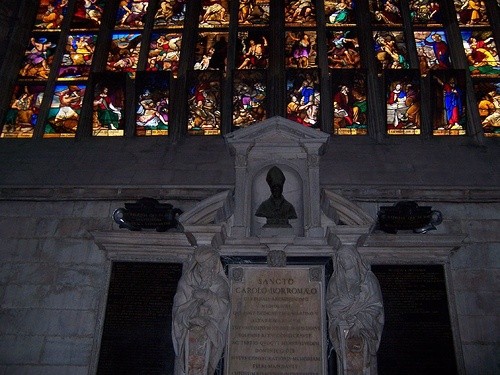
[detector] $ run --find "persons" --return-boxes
[255,176,297,218]
